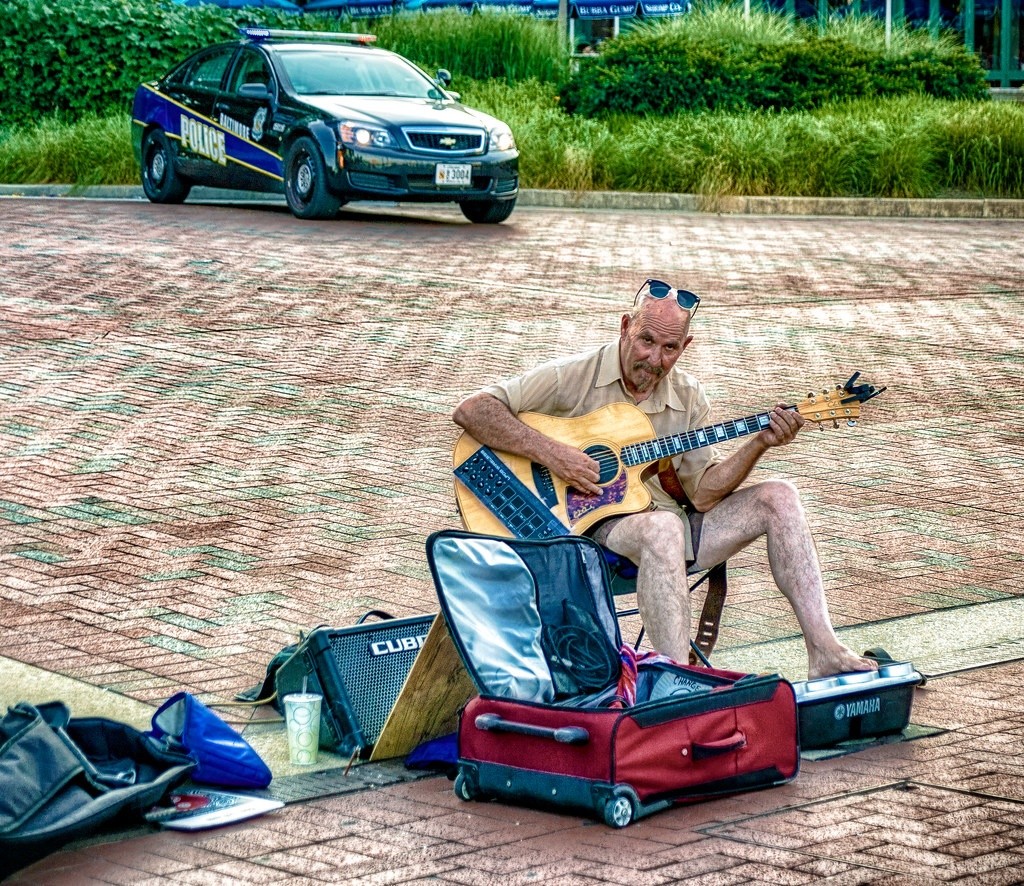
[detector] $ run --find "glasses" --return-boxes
[634,278,701,320]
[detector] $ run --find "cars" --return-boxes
[130,28,521,223]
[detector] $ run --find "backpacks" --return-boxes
[0,697,198,847]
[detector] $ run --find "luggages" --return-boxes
[423,529,802,826]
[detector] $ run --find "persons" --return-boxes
[452,279,878,680]
[578,43,593,53]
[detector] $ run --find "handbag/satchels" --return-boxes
[141,691,273,791]
[232,623,340,749]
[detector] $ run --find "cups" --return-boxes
[283,693,322,765]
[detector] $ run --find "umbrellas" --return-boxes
[301,0,405,34]
[171,0,302,16]
[405,0,690,77]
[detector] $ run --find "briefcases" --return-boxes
[308,609,439,763]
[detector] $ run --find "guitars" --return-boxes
[448,366,892,545]
